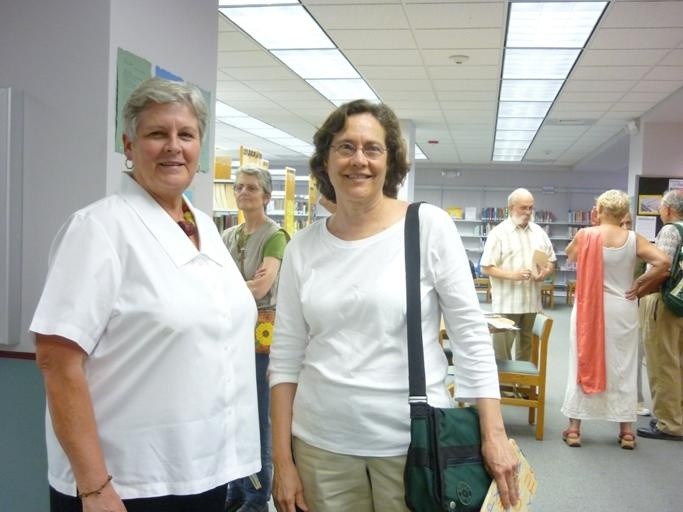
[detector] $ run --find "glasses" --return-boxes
[330,143,388,157]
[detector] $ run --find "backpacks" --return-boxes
[662,221,683,317]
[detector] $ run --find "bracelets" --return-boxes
[72,475,118,499]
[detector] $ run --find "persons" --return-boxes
[637,186,683,440]
[559,188,673,450]
[618,208,652,417]
[215,165,311,511]
[262,98,523,511]
[27,75,266,511]
[478,185,557,397]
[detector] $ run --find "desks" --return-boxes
[439,316,516,337]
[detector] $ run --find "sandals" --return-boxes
[562,429,582,447]
[617,431,636,448]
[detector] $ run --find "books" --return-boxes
[213,197,309,235]
[477,436,538,512]
[530,248,549,277]
[477,207,590,293]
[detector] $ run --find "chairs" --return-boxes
[496,312,553,441]
[439,329,465,408]
[542,264,576,307]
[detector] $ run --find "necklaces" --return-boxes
[175,203,196,237]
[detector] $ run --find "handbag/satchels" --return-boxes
[404,405,497,512]
[254,306,278,357]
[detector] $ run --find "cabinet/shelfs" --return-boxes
[452,219,591,295]
[212,145,319,233]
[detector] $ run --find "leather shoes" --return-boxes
[638,404,652,417]
[636,425,683,441]
[648,416,658,427]
